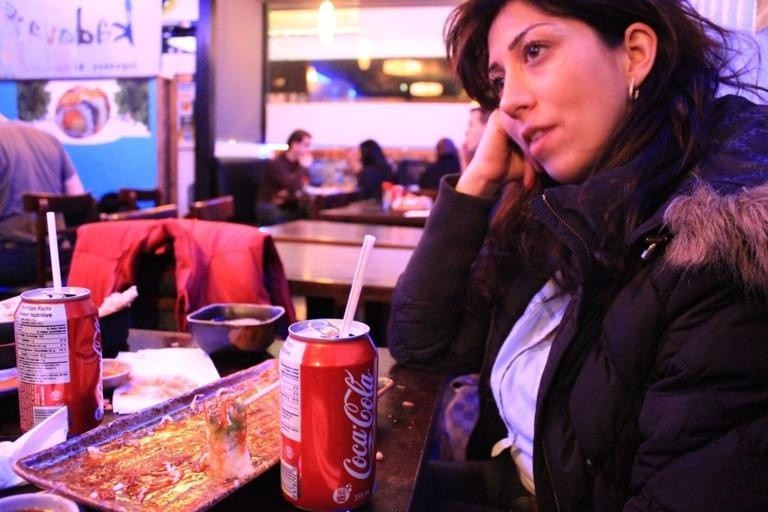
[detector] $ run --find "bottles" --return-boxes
[378,182,394,213]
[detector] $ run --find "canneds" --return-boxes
[13,285,105,439]
[278,318,380,511]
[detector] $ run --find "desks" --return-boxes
[1,158,507,511]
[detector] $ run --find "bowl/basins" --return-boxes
[0,358,130,425]
[10,359,391,510]
[185,302,289,356]
[1,491,80,511]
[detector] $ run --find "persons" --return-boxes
[254,106,491,228]
[380,1,768,511]
[0,111,85,289]
